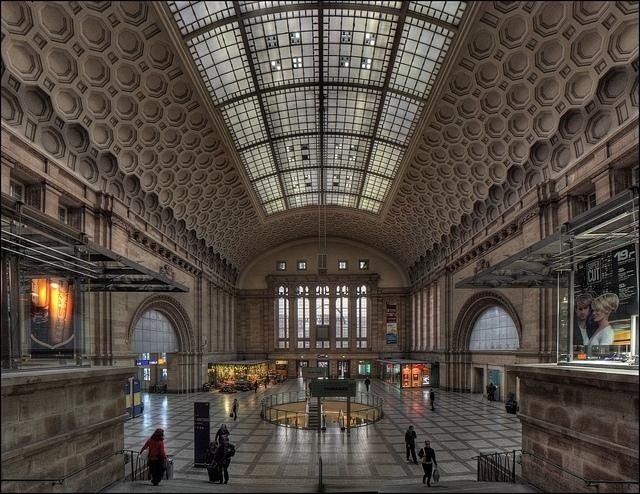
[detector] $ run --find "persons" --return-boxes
[162,455,169,480]
[232,399,239,421]
[253,374,269,393]
[487,383,496,401]
[206,424,235,484]
[573,293,598,346]
[418,440,437,487]
[146,454,151,481]
[365,377,370,392]
[405,425,418,465]
[139,428,168,486]
[586,293,620,345]
[430,389,435,411]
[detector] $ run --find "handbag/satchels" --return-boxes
[433,469,440,482]
[419,453,426,463]
[230,412,233,417]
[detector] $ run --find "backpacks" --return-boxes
[228,445,235,457]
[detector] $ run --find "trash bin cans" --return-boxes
[166,458,173,480]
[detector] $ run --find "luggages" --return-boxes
[205,443,218,465]
[207,464,220,484]
